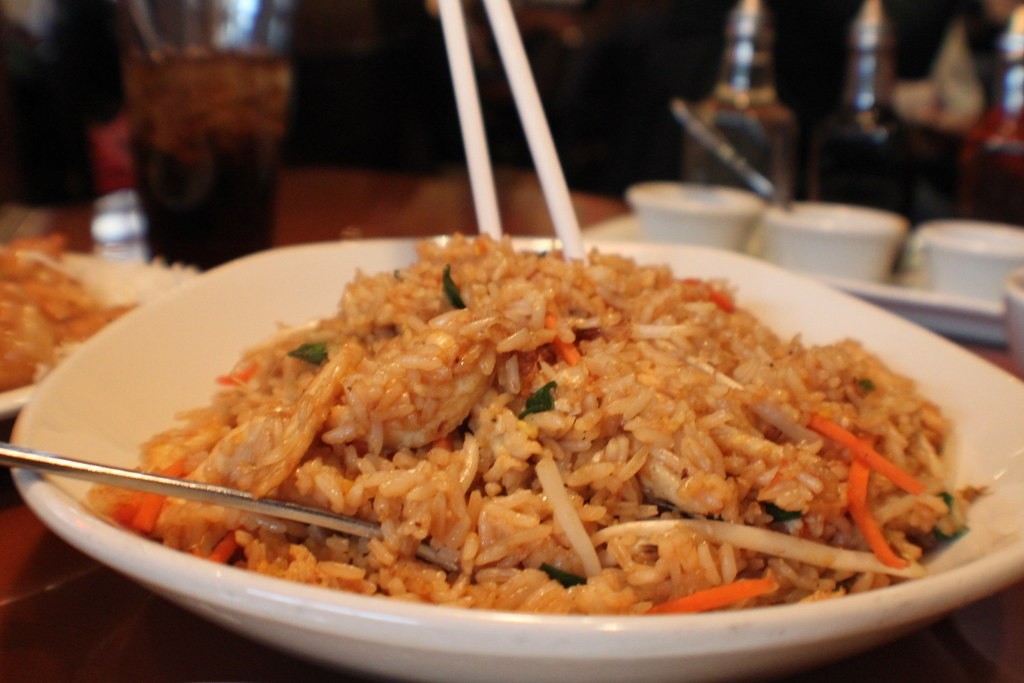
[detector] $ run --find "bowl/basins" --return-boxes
[763,201,906,284]
[1003,269,1024,375]
[626,182,761,252]
[906,219,1023,299]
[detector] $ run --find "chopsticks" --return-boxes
[438,0,586,259]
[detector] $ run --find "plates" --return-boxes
[0,383,33,420]
[9,236,1024,682]
[581,216,1004,342]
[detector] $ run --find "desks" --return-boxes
[0,165,1024,683]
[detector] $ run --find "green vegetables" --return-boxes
[283,261,558,419]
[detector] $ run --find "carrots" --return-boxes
[643,579,779,615]
[843,435,910,568]
[808,414,922,494]
[110,460,244,566]
[543,314,580,364]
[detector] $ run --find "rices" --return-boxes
[123,232,975,615]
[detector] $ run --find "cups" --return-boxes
[117,1,292,270]
